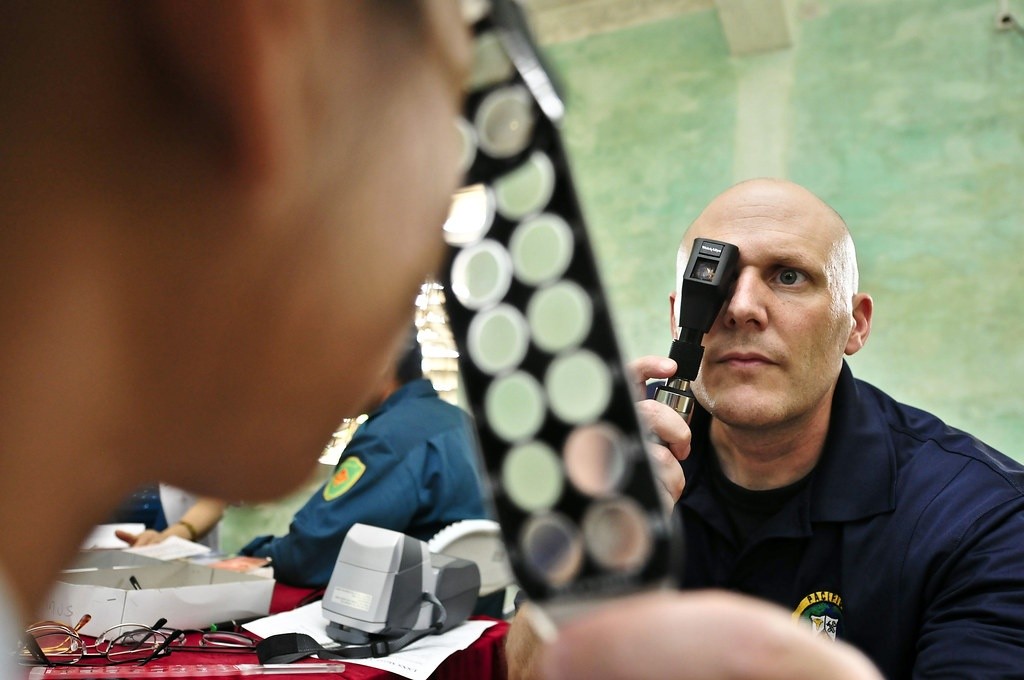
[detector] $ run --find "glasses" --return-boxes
[15,614,183,667]
[121,617,257,650]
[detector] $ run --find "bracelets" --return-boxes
[177,520,196,540]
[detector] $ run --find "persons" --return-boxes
[230,321,504,587]
[101,479,225,548]
[523,177,1024,680]
[0,1,509,679]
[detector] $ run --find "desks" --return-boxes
[12,581,509,680]
